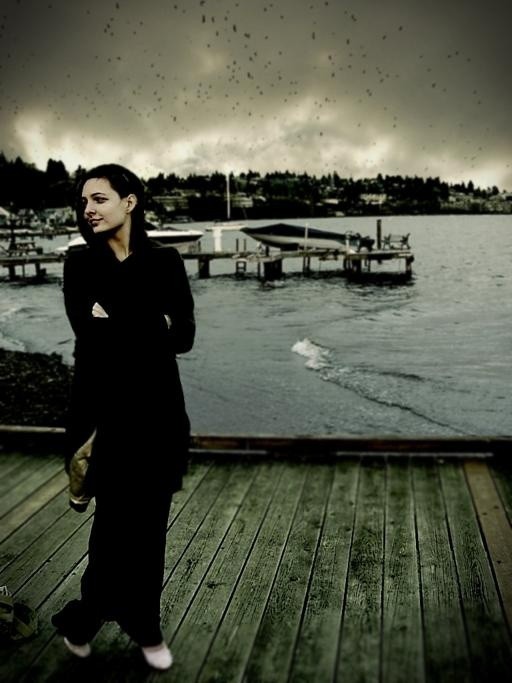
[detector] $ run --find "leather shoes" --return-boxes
[63,634,92,658]
[141,640,174,671]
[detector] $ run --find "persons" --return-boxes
[51,164,195,670]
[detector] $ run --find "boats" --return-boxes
[67,226,204,248]
[204,222,241,232]
[242,222,375,250]
